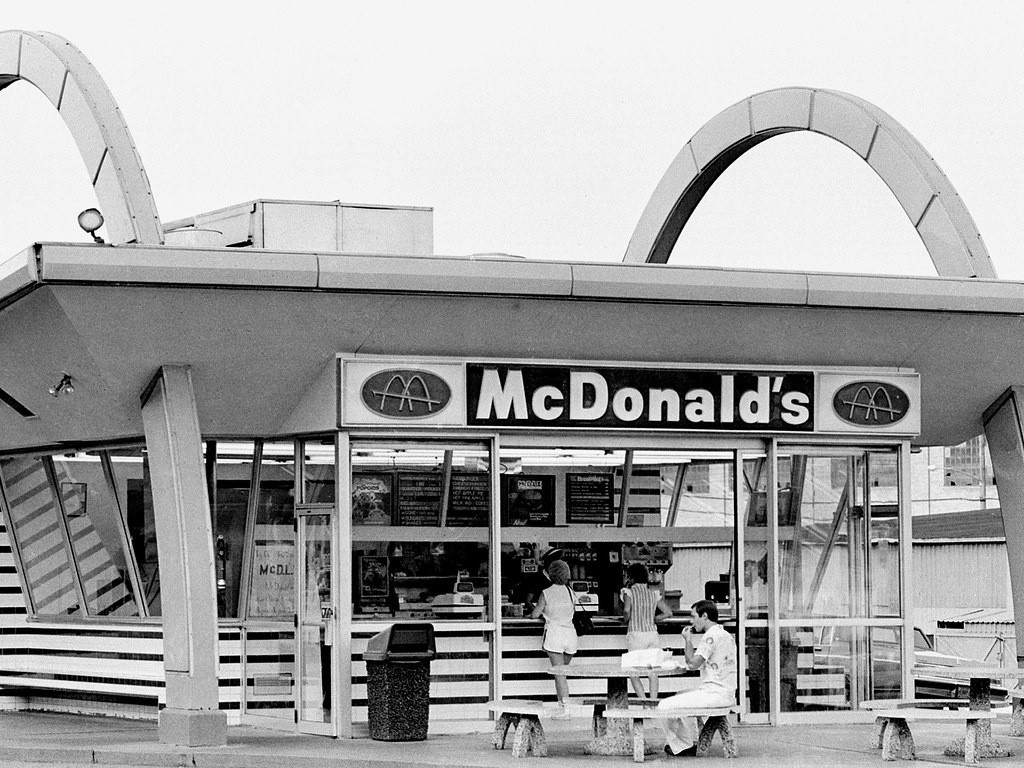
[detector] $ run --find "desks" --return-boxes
[911,667,1024,758]
[547,663,686,756]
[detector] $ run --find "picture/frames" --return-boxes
[61,482,87,517]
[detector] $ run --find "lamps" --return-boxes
[77,208,106,243]
[46,373,74,397]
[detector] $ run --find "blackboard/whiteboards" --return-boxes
[360,555,390,598]
[446,472,491,527]
[394,470,442,526]
[565,472,615,524]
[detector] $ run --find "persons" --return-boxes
[658,600,737,756]
[534,546,578,721]
[620,563,672,709]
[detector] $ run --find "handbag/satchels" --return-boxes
[567,585,596,637]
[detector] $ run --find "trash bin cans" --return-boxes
[362,623,437,742]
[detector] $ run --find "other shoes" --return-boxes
[664,744,698,757]
[550,706,572,720]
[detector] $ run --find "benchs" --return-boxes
[859,699,1024,766]
[485,696,741,762]
